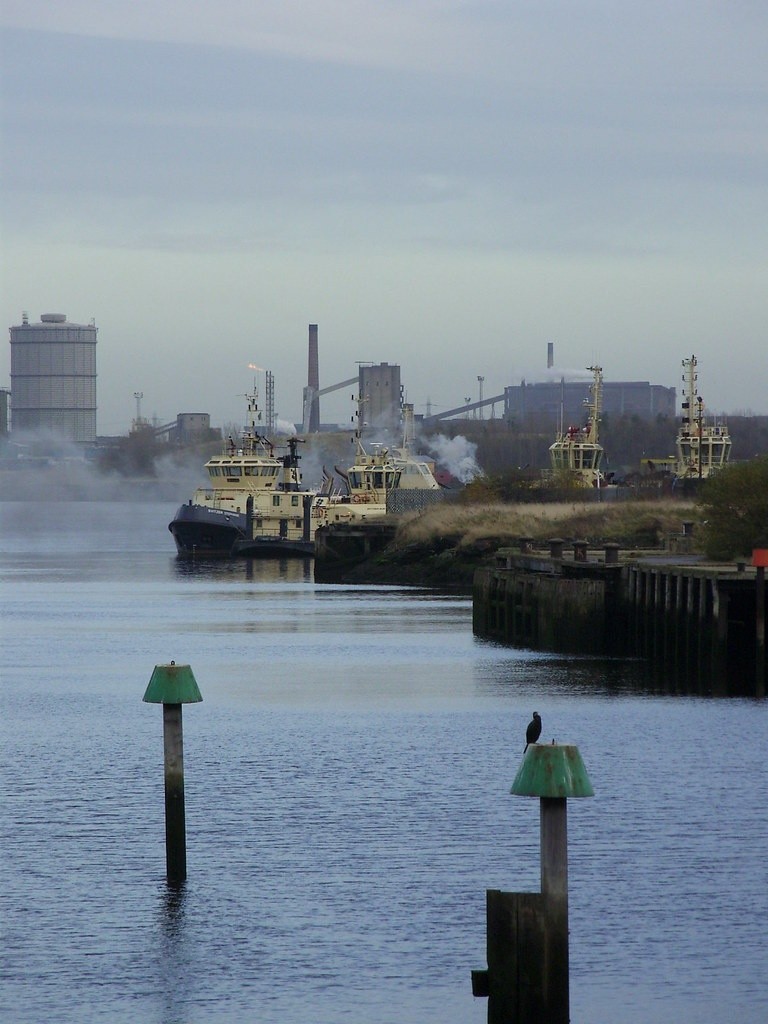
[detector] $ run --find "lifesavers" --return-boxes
[353,495,361,503]
[317,507,324,517]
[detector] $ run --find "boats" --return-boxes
[162,375,442,561]
[658,352,736,488]
[526,365,628,492]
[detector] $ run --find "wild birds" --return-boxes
[523,710,542,754]
